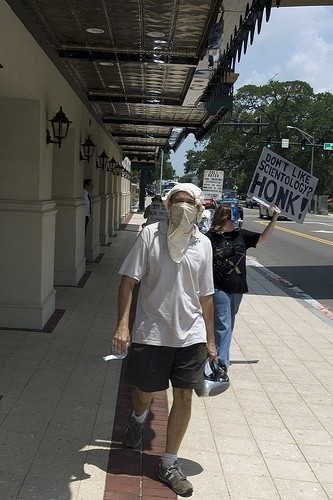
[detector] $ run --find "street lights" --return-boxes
[286,125,315,213]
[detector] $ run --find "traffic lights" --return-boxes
[266,136,271,149]
[301,139,305,151]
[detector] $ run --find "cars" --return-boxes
[203,197,238,209]
[245,197,260,209]
[259,202,294,221]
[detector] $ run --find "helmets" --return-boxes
[194,358,230,399]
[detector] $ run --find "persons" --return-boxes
[110,181,218,496]
[143,192,279,383]
[82,179,94,236]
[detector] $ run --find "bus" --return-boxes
[161,180,179,196]
[222,189,238,199]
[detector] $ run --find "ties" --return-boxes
[87,194,91,215]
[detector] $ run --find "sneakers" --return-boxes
[123,408,149,449]
[157,461,193,494]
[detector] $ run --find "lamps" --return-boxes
[107,156,132,180]
[80,135,97,164]
[96,148,109,172]
[46,105,73,149]
